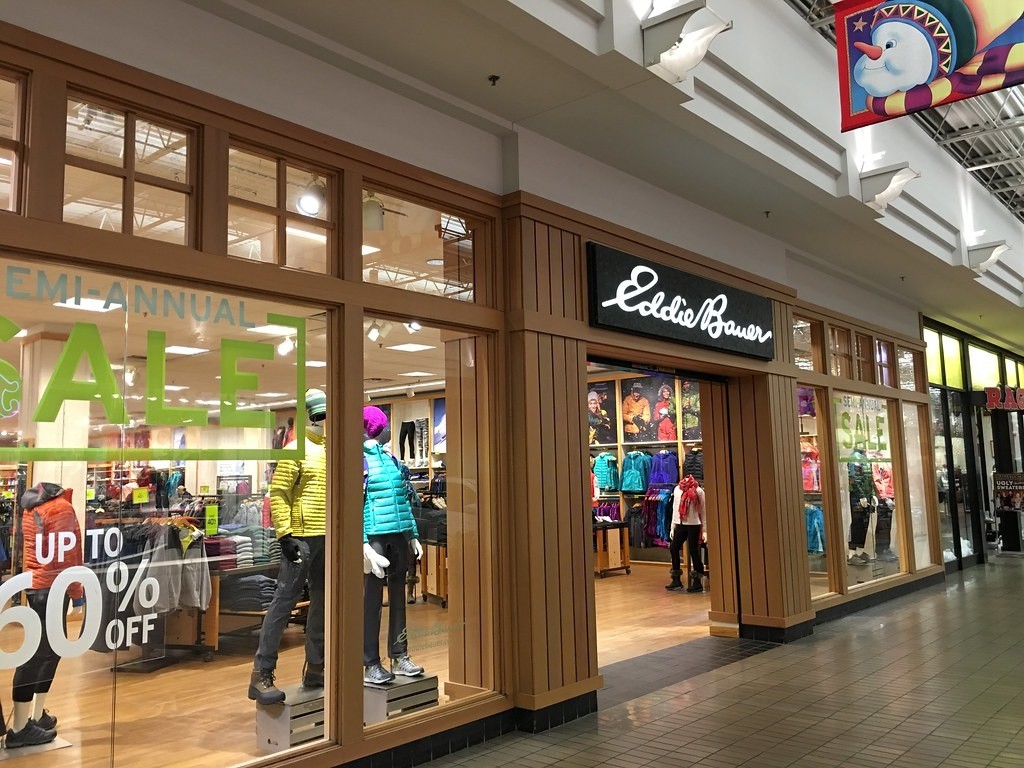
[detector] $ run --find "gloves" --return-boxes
[634,414,645,426]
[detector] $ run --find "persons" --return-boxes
[654,385,675,441]
[270,418,294,468]
[846,446,878,566]
[248,388,326,705]
[588,391,610,444]
[415,418,428,467]
[5,483,84,747]
[363,406,425,683]
[668,381,701,440]
[996,490,1024,512]
[176,485,191,498]
[871,450,896,562]
[665,475,708,592]
[399,421,415,466]
[171,434,185,467]
[622,386,651,442]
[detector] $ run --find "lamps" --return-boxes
[641,0,733,84]
[296,172,442,342]
[407,388,414,397]
[364,393,371,402]
[967,240,1012,273]
[859,161,921,209]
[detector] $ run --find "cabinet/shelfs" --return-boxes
[589,440,708,568]
[1,468,16,494]
[363,394,446,493]
[87,463,130,495]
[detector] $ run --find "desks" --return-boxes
[165,562,310,662]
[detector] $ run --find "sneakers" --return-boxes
[247,668,285,705]
[27,709,59,730]
[390,657,425,677]
[363,663,396,684]
[845,554,867,565]
[876,550,899,563]
[687,581,703,592]
[304,662,324,687]
[858,552,876,563]
[5,719,57,749]
[665,580,684,591]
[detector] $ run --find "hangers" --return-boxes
[803,498,811,509]
[801,437,813,450]
[633,498,643,507]
[598,442,703,456]
[601,498,620,507]
[142,510,194,531]
[645,483,669,501]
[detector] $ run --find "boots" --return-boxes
[406,576,420,604]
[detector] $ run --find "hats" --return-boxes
[590,382,609,391]
[305,387,327,417]
[588,391,599,404]
[364,405,388,440]
